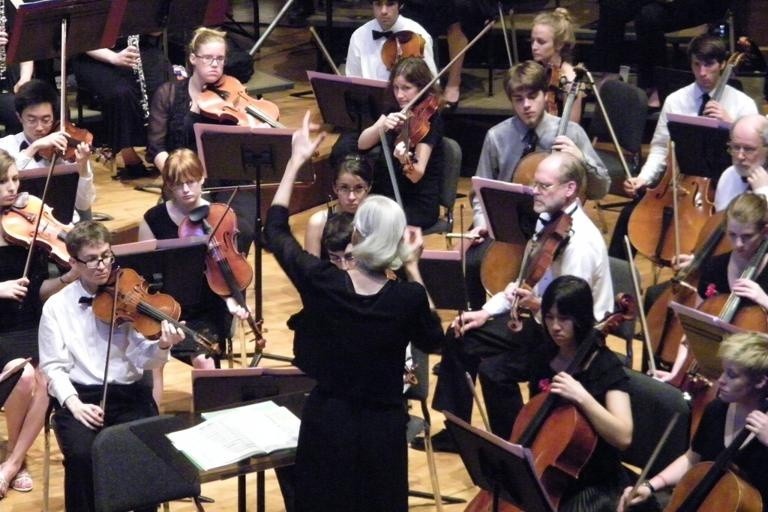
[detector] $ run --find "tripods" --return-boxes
[220,164,294,368]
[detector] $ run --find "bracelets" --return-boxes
[60,278,70,284]
[645,480,656,493]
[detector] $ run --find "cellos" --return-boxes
[479,72,590,296]
[626,35,751,265]
[666,239,768,447]
[663,401,768,512]
[646,160,768,360]
[463,292,637,511]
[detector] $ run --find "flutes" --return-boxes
[0,2,8,92]
[127,34,150,130]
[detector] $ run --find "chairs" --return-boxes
[550,363,694,487]
[579,77,652,235]
[404,343,447,512]
[88,410,208,512]
[396,134,463,252]
[569,253,642,372]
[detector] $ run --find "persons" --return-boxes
[1,149,81,498]
[38,221,185,512]
[465,62,612,313]
[263,110,443,512]
[412,152,614,453]
[607,32,768,263]
[148,28,256,257]
[618,332,767,512]
[345,1,731,124]
[0,81,95,224]
[139,148,252,370]
[1,0,253,168]
[345,56,447,229]
[647,193,767,386]
[529,274,633,511]
[276,155,414,507]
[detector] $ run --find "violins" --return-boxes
[508,213,572,332]
[540,63,559,118]
[393,96,439,171]
[1,191,76,269]
[179,203,268,350]
[381,31,424,69]
[92,266,223,356]
[38,120,113,164]
[197,73,285,129]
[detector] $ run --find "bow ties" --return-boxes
[20,140,43,162]
[373,30,394,41]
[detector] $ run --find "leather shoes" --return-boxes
[412,428,460,455]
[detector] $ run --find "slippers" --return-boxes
[0,461,10,500]
[8,471,33,492]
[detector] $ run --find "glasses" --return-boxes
[195,55,228,66]
[334,182,372,199]
[21,115,54,128]
[727,146,757,160]
[73,250,115,269]
[529,179,556,194]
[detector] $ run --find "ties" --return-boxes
[698,93,710,116]
[510,131,539,182]
